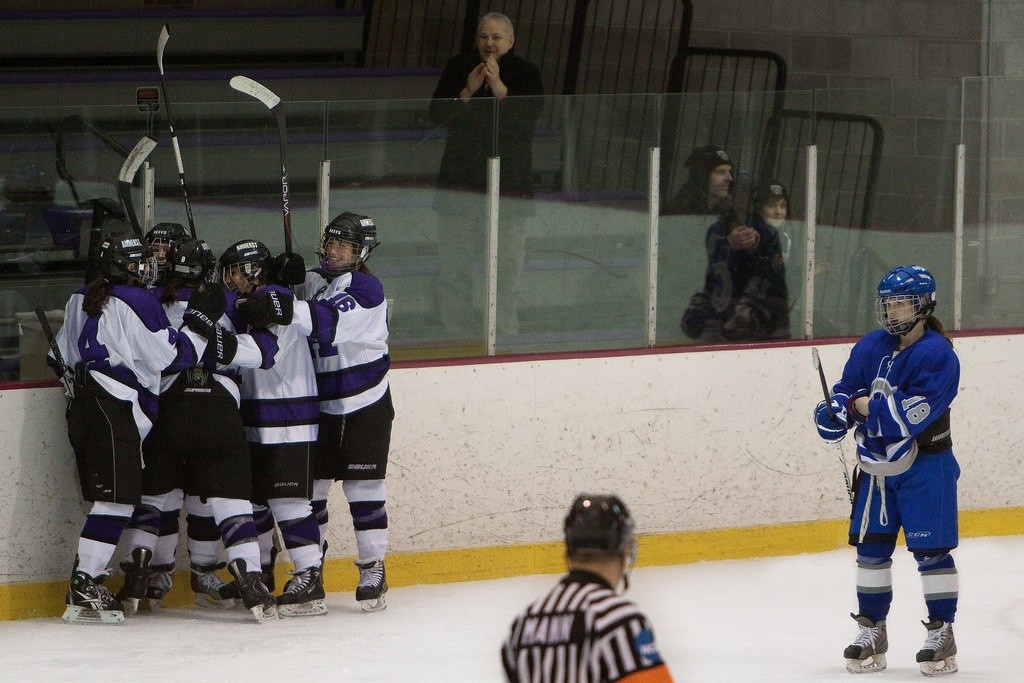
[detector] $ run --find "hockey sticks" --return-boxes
[228,74,294,261]
[117,135,159,239]
[156,23,197,244]
[812,347,855,506]
[34,304,79,401]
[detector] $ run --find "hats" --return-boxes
[755,181,788,204]
[685,146,733,186]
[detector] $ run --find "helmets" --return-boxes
[564,494,634,554]
[319,214,378,276]
[877,266,936,335]
[217,239,272,296]
[98,224,214,288]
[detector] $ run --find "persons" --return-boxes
[813,264,961,676]
[430,12,544,338]
[502,492,675,683]
[48,212,397,623]
[654,145,793,345]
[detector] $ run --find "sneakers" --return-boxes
[355,556,388,612]
[844,612,889,673]
[219,545,278,625]
[187,548,236,613]
[115,547,175,617]
[275,539,328,619]
[916,617,958,677]
[62,553,125,622]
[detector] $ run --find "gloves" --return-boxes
[814,393,855,444]
[846,388,870,426]
[272,253,306,286]
[206,323,238,365]
[234,292,294,326]
[182,284,227,339]
